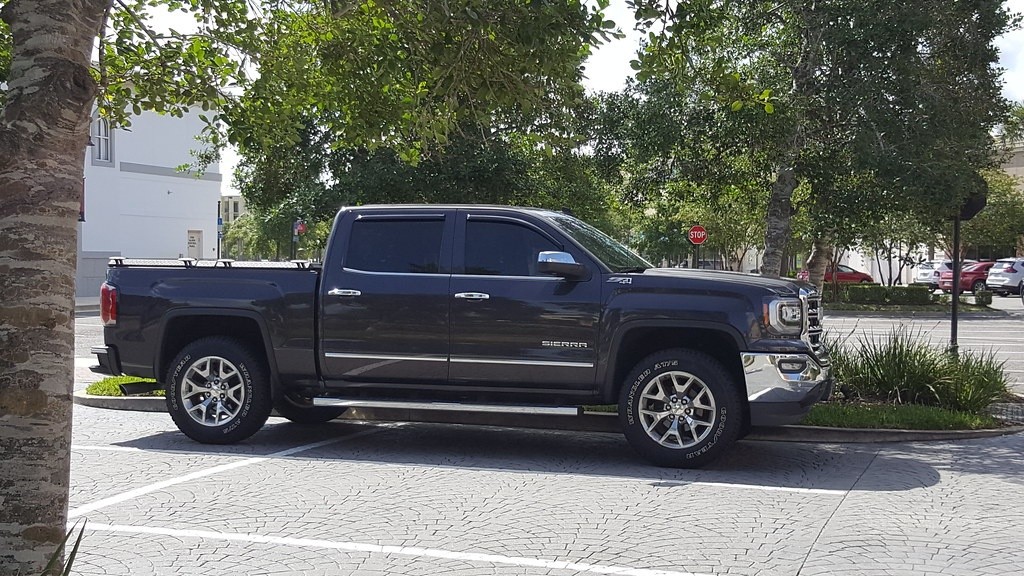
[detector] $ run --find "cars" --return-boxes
[796,264,874,283]
[939,261,996,294]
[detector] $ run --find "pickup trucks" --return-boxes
[88,204,835,470]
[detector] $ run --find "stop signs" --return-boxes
[687,225,708,245]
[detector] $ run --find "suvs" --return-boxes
[912,258,980,294]
[986,257,1024,298]
